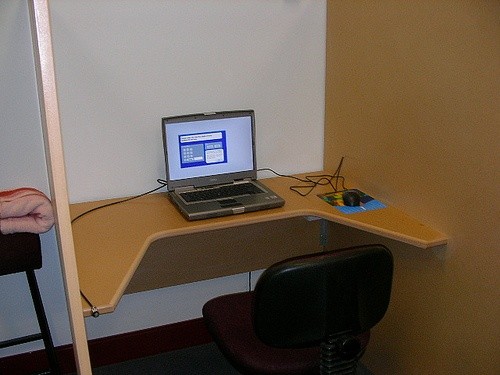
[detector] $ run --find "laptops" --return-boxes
[161,109,285,220]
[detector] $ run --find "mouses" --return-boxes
[343,191,361,207]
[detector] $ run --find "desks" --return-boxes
[0,233,59,375]
[69,168,450,318]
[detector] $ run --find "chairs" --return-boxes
[201,244,395,375]
